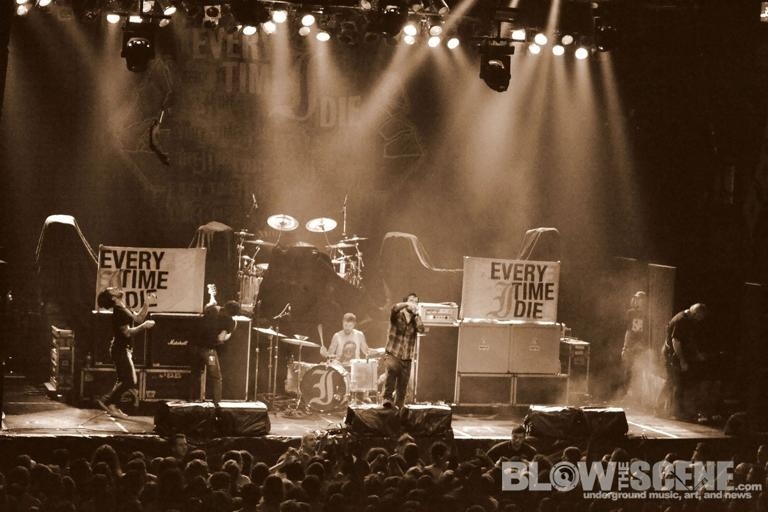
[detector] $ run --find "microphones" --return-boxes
[342,193,348,214]
[250,192,259,212]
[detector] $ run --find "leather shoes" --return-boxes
[383,399,391,407]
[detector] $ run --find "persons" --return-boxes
[0,407,768,511]
[652,302,710,423]
[186,296,241,406]
[319,312,385,405]
[95,285,158,421]
[382,291,426,410]
[619,290,650,409]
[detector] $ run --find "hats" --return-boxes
[359,474,385,490]
[252,463,270,478]
[98,445,118,460]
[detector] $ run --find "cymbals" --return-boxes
[369,347,386,357]
[341,237,367,241]
[281,336,320,349]
[305,218,338,233]
[325,244,355,249]
[266,214,299,232]
[253,327,286,338]
[243,239,274,247]
[233,231,255,236]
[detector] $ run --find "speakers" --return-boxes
[143,313,207,375]
[560,337,591,394]
[91,310,145,369]
[412,323,459,406]
[139,368,207,403]
[203,315,252,403]
[460,373,514,409]
[154,399,271,439]
[80,366,143,402]
[529,403,628,437]
[345,403,455,441]
[516,374,570,406]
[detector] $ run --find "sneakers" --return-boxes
[98,400,110,411]
[109,408,128,417]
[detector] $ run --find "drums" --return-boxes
[332,259,355,277]
[343,358,378,392]
[297,358,353,413]
[285,363,315,393]
[237,272,270,314]
[254,263,268,276]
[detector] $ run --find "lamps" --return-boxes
[470,17,516,94]
[16,0,617,92]
[120,10,159,75]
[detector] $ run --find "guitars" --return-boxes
[205,284,235,341]
